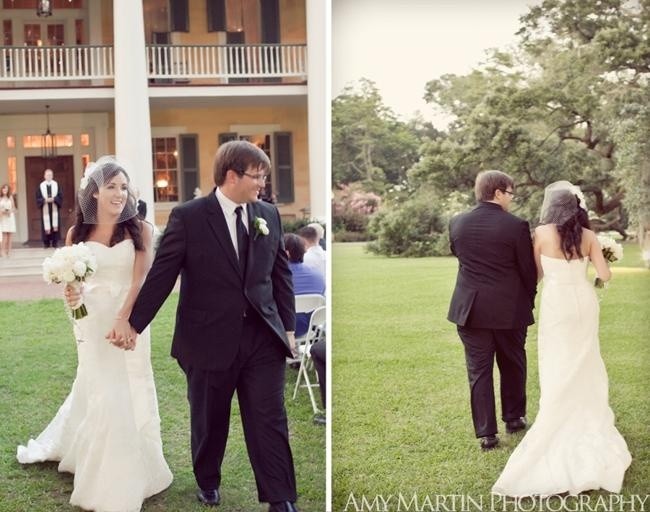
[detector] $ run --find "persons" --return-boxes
[489,179,632,500]
[35,169,63,249]
[105,139,298,510]
[15,154,174,511]
[0,183,17,258]
[445,170,538,449]
[130,188,147,221]
[281,223,326,426]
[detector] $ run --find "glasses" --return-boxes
[243,171,268,181]
[503,190,514,199]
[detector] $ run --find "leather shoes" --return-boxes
[197,489,221,504]
[480,435,500,449]
[313,408,326,423]
[506,416,527,432]
[269,500,297,512]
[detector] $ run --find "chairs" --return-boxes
[292,309,326,416]
[285,295,326,366]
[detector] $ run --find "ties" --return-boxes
[233,205,249,282]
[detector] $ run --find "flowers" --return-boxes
[333,183,380,221]
